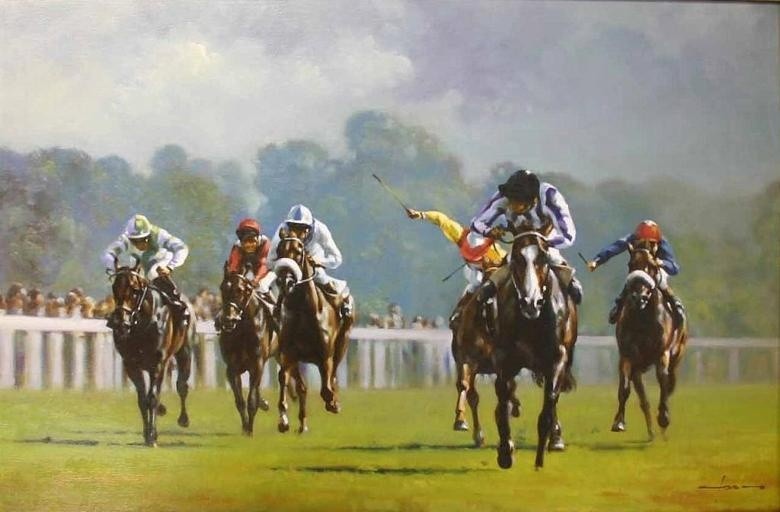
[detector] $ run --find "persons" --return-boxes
[1,282,222,320]
[407,208,510,298]
[588,220,685,327]
[366,301,443,328]
[215,218,276,332]
[471,170,584,305]
[266,203,352,320]
[100,213,189,329]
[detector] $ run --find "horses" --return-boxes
[104,252,193,449]
[487,214,585,472]
[607,239,690,440]
[448,250,522,448]
[213,260,298,439]
[272,228,356,436]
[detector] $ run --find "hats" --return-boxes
[235,218,261,236]
[634,219,664,248]
[125,214,153,239]
[285,204,314,227]
[497,169,540,199]
[461,230,493,261]
